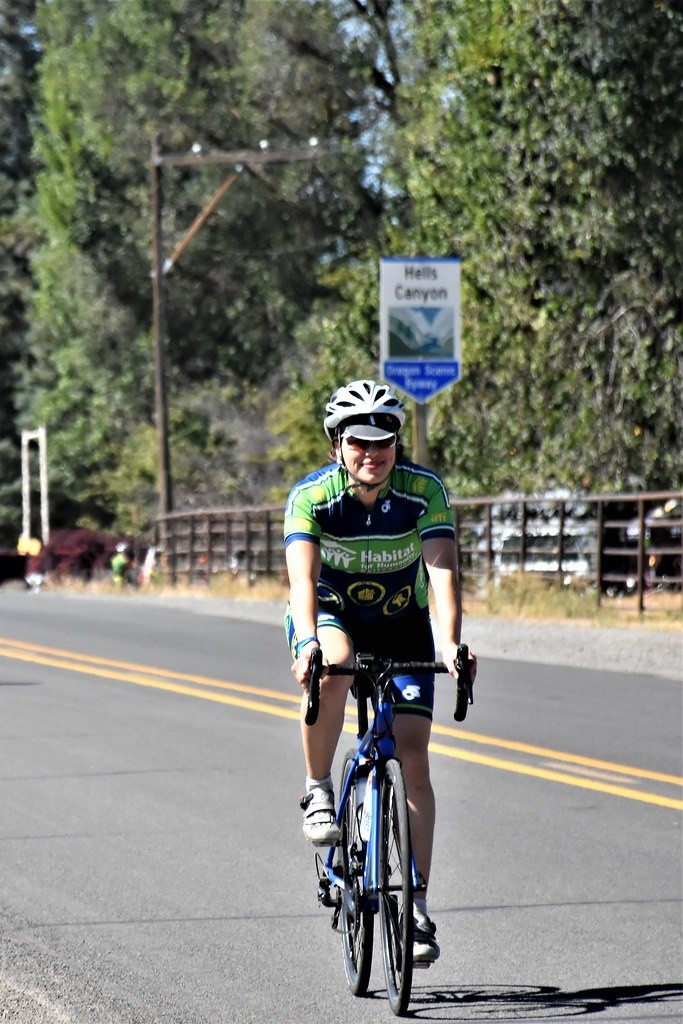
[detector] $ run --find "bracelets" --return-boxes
[297,637,321,654]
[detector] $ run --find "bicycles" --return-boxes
[305,645,477,1018]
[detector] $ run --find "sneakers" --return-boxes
[303,782,340,843]
[412,909,440,961]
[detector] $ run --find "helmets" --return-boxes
[323,380,405,443]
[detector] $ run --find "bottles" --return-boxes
[360,769,373,842]
[353,765,371,852]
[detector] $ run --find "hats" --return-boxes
[332,414,401,448]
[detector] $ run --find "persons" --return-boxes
[282,380,478,963]
[111,541,167,589]
